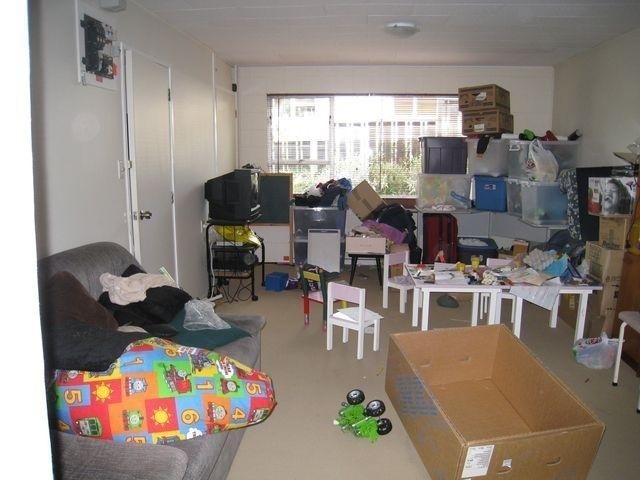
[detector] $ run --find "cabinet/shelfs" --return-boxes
[205,222,265,301]
[349,235,387,282]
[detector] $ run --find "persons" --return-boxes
[600,179,631,215]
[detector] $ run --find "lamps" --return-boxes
[384,22,421,37]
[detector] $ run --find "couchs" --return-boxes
[38,241,267,480]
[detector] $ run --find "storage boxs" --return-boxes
[349,179,387,220]
[460,84,511,111]
[461,106,515,137]
[471,173,509,212]
[418,136,469,174]
[506,179,523,216]
[509,134,580,182]
[294,238,347,272]
[583,240,625,285]
[558,294,605,338]
[295,206,345,239]
[465,137,509,177]
[520,180,570,230]
[415,173,473,213]
[384,323,607,480]
[597,215,631,249]
[570,284,620,317]
[265,271,289,292]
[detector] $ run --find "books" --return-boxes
[434,270,468,287]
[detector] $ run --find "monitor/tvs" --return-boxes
[205,170,260,221]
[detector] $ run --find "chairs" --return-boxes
[299,266,349,330]
[381,250,414,314]
[326,283,381,359]
[611,309,640,414]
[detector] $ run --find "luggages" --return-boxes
[372,203,416,242]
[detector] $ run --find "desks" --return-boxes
[404,262,603,344]
